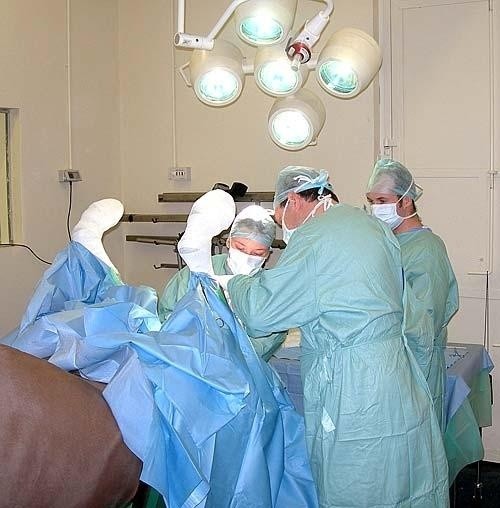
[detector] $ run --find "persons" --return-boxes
[71,190,236,316]
[156,206,286,365]
[213,166,453,508]
[365,159,457,428]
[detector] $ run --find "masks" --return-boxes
[226,235,271,276]
[370,195,413,231]
[281,191,308,246]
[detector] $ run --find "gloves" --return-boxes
[213,275,235,290]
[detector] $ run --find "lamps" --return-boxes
[174,0,382,152]
